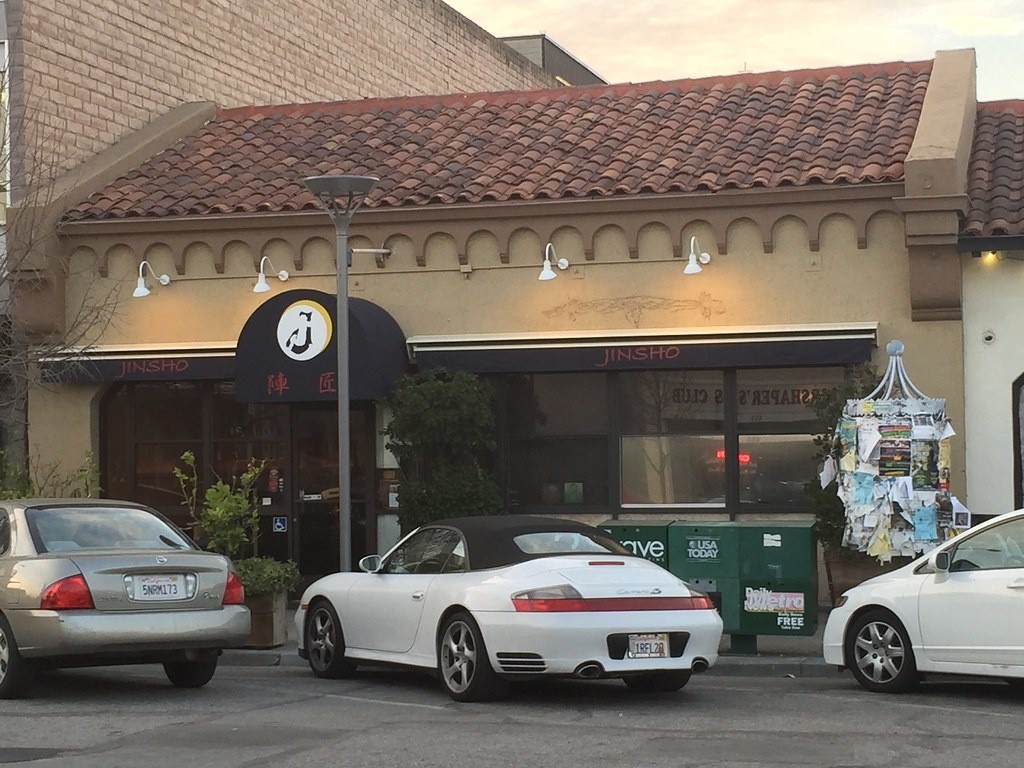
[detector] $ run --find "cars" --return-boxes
[827,507,1024,695]
[0,497,252,699]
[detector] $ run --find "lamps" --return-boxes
[683,235,711,275]
[252,255,290,293]
[132,261,171,298]
[537,242,569,281]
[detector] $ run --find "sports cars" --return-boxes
[292,513,726,706]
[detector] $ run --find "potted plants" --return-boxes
[231,553,300,649]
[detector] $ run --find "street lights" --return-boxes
[305,175,380,576]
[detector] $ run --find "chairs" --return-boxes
[114,541,160,548]
[45,541,81,551]
